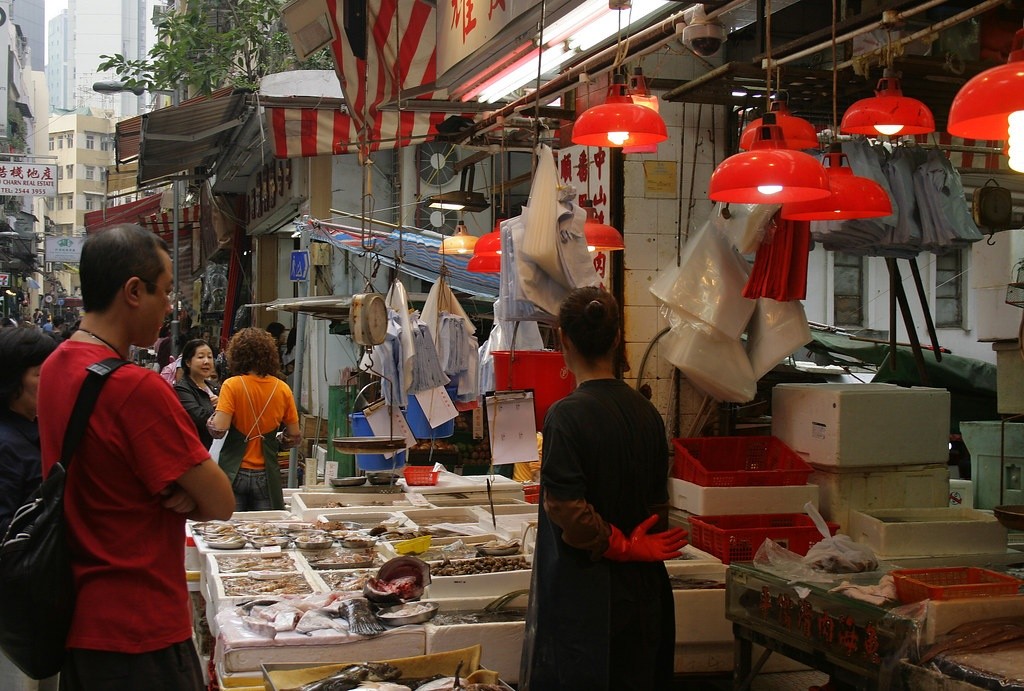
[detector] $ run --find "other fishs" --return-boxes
[202,507,507,691]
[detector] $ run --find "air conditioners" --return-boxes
[399,140,496,238]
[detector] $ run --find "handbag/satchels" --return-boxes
[1,494,73,681]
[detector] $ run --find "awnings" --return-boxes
[138,205,201,241]
[302,220,500,298]
[83,193,162,235]
[26,277,40,289]
[105,84,242,192]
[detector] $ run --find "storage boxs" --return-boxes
[993,341,1024,422]
[183,385,1024,691]
[971,229,1024,343]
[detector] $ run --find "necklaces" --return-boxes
[76,329,125,361]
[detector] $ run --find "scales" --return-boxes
[330,194,407,455]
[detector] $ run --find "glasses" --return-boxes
[143,280,182,305]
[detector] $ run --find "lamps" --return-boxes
[423,0,1024,273]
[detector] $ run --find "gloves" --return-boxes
[607,514,690,562]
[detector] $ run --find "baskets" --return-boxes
[888,565,1024,604]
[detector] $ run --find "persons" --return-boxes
[174,321,302,512]
[154,326,169,354]
[33,307,81,343]
[0,325,59,539]
[517,286,689,691]
[37,224,236,691]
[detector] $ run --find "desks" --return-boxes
[732,598,1024,691]
